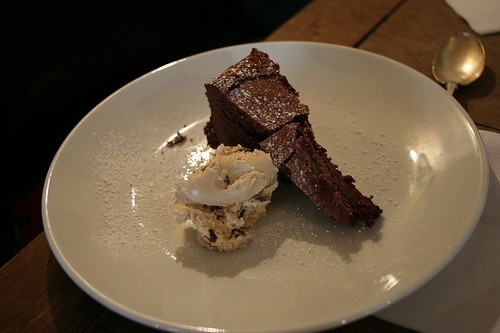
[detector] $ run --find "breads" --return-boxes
[205,48,384,228]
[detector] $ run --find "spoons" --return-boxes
[432,30,486,96]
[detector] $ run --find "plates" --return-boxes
[41,41,490,333]
